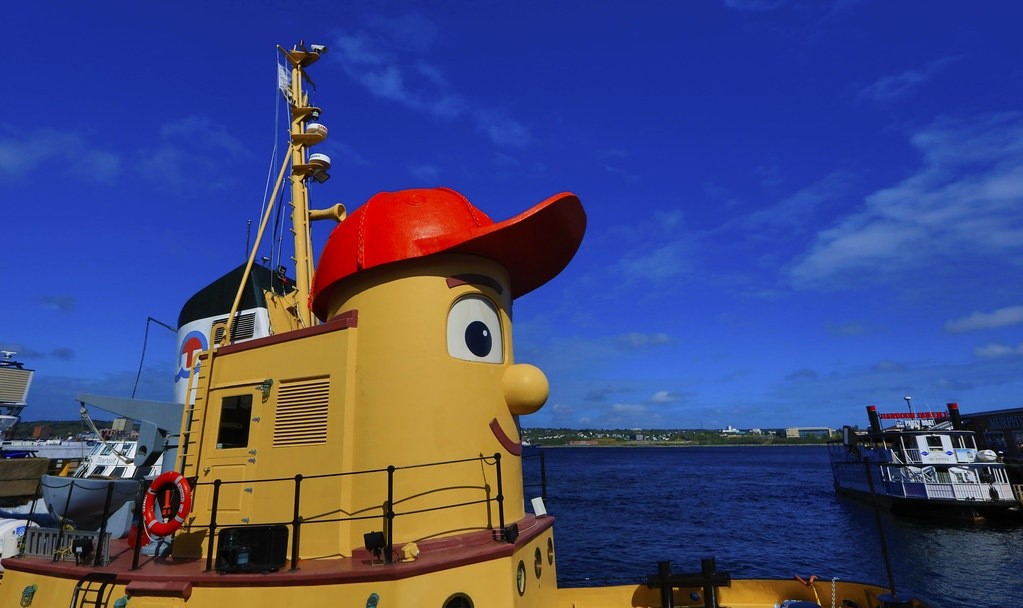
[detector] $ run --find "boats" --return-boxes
[830,396,1023,524]
[8,48,924,608]
[41,402,183,529]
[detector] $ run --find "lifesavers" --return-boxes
[141,471,192,537]
